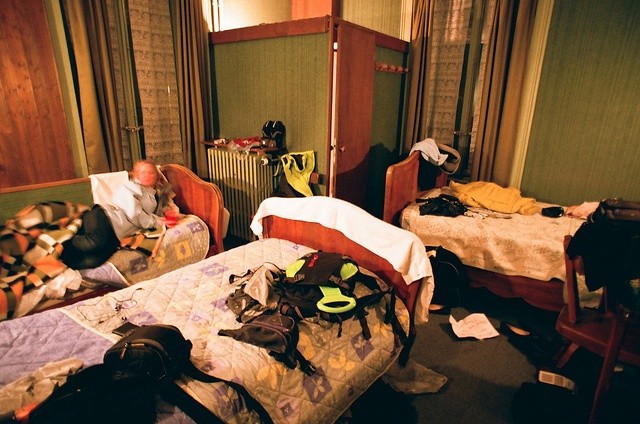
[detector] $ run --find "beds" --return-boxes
[383,143,640,311]
[0,195,435,423]
[1,164,230,320]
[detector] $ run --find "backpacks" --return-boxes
[285,251,410,348]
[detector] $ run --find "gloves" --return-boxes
[224,289,271,324]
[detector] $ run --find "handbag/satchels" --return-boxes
[418,194,469,218]
[103,325,274,424]
[218,313,317,378]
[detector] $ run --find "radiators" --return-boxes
[208,147,277,241]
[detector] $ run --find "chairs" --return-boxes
[556,234,639,424]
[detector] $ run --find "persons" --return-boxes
[58,159,178,269]
